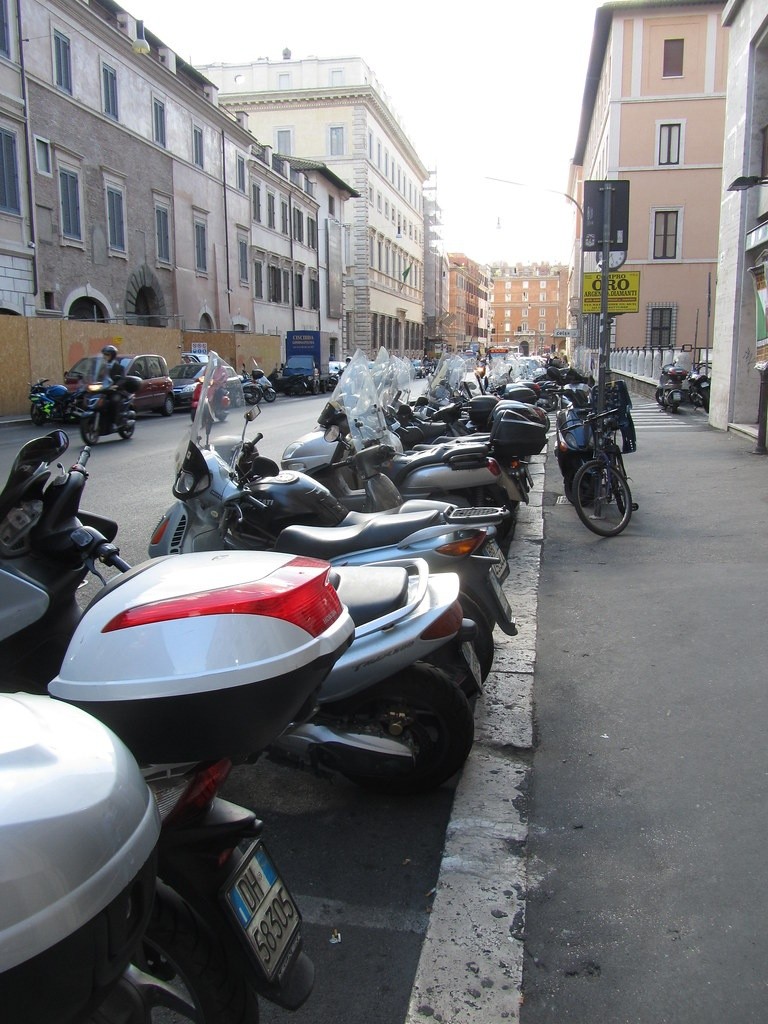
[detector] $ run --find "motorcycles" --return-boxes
[241,362,276,405]
[79,352,136,447]
[655,360,710,413]
[327,366,355,394]
[27,378,90,426]
[0,346,558,1024]
[554,409,617,505]
[266,362,312,397]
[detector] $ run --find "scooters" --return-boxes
[191,373,231,422]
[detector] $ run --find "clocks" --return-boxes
[596,250,627,271]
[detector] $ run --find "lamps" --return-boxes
[727,175,768,191]
[395,226,402,238]
[132,19,151,54]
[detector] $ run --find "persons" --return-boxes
[193,351,228,411]
[341,357,351,373]
[97,345,126,430]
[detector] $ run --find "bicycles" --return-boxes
[561,407,638,537]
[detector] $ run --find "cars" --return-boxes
[63,354,176,417]
[159,352,250,408]
[329,359,426,388]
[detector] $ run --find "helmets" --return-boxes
[102,345,118,358]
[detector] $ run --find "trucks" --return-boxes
[281,330,329,395]
